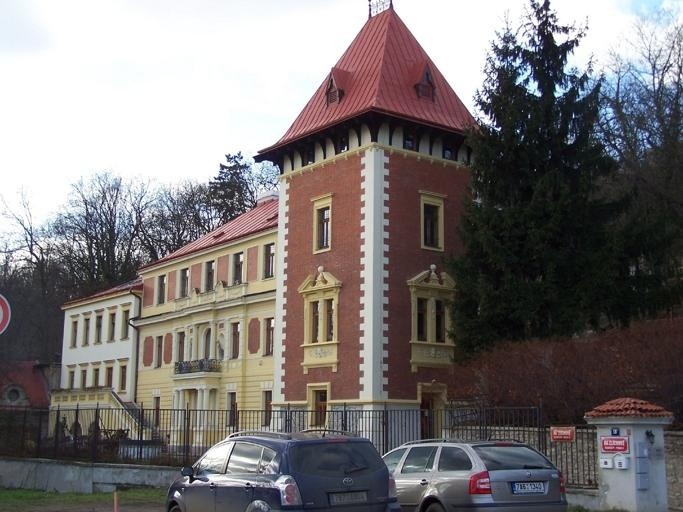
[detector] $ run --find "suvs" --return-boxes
[167,427,399,512]
[380,436,569,512]
